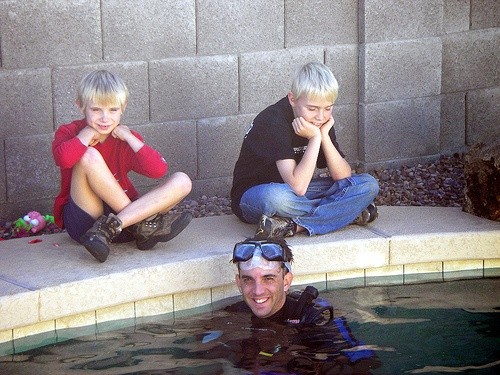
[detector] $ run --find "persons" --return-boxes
[229,62,380,239]
[54,70,194,264]
[225,235,333,325]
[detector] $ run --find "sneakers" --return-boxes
[80,212,123,262]
[254,215,296,239]
[136,213,193,250]
[350,204,378,225]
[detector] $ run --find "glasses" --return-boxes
[233,242,292,273]
